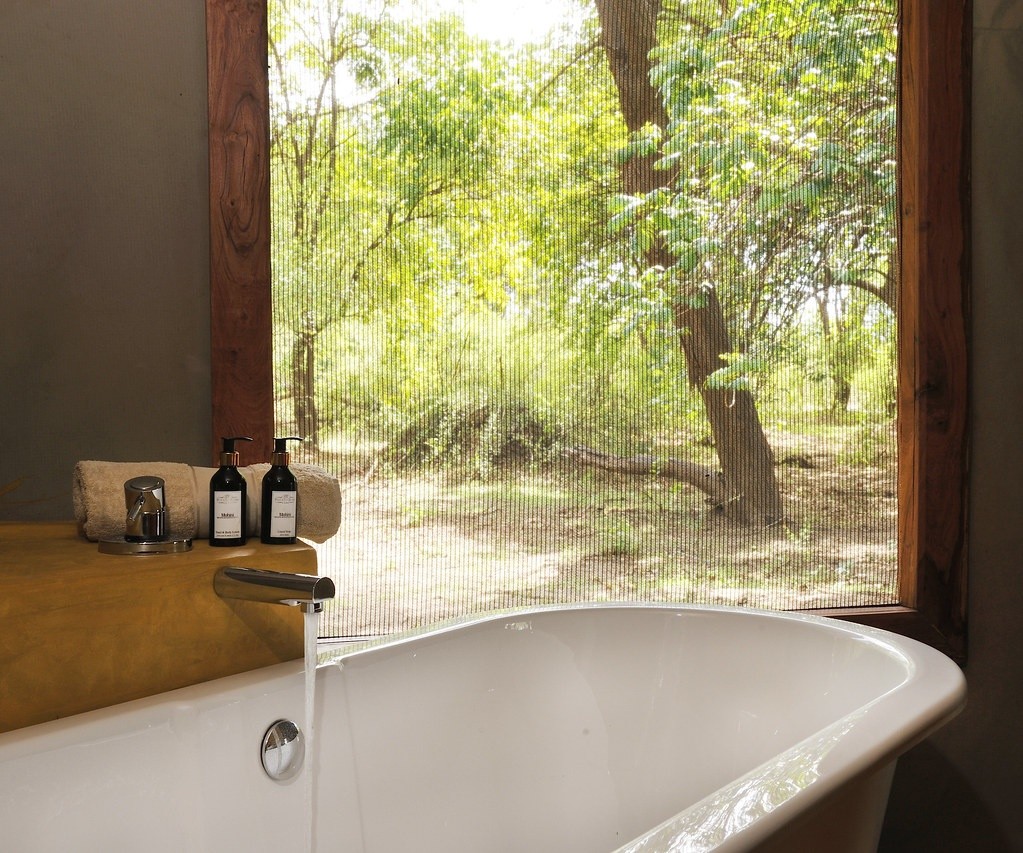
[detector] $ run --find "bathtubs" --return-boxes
[0,599,970,853]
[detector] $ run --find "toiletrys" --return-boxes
[208,435,255,548]
[259,436,304,546]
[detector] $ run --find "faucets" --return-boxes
[213,564,337,614]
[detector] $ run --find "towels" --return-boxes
[71,459,342,544]
